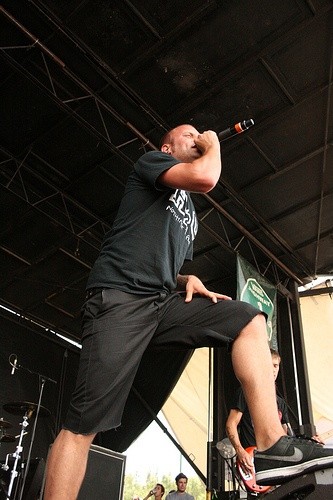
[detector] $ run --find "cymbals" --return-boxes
[3,401,51,417]
[0,434,17,443]
[0,421,13,429]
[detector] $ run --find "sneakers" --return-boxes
[253,434,333,485]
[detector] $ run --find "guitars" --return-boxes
[236,429,333,493]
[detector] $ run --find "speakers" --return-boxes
[258,468,333,500]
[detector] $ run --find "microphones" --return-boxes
[12,358,17,374]
[218,119,255,142]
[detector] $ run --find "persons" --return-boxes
[44,125,333,500]
[225,348,325,500]
[165,473,194,500]
[143,483,165,500]
